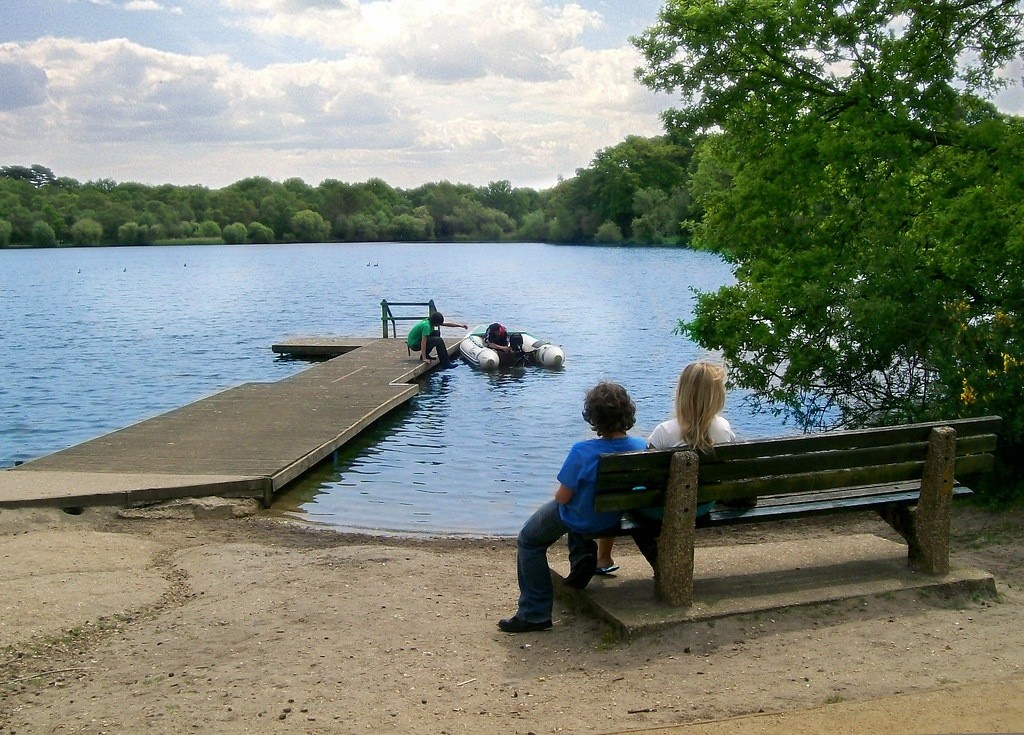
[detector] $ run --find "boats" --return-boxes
[460,321,566,371]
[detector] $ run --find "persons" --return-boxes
[485,322,513,354]
[407,312,468,369]
[595,361,737,574]
[496,379,649,633]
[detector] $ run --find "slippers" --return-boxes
[594,566,620,574]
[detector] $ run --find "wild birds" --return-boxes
[367,262,378,266]
[122,267,126,272]
[183,262,187,268]
[78,268,81,274]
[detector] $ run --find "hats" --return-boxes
[500,326,506,335]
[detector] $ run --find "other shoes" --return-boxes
[443,363,459,370]
[420,353,437,360]
[498,615,553,632]
[563,555,597,587]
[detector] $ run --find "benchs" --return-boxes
[597,414,1004,606]
